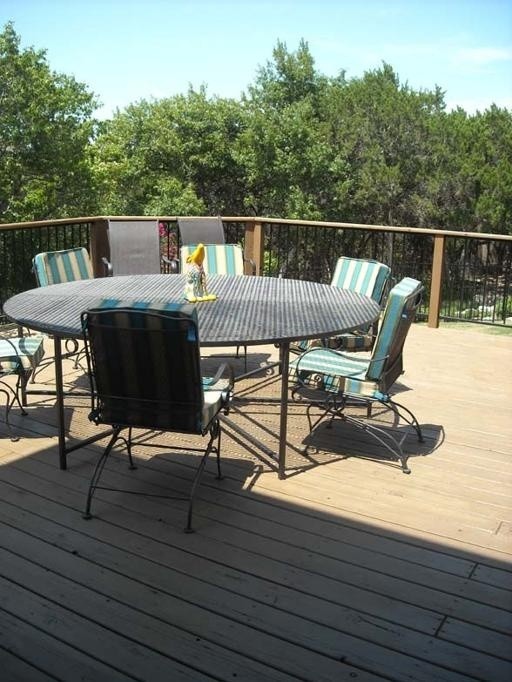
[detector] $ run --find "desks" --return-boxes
[1,277,382,480]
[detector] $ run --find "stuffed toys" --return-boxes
[186,243,216,303]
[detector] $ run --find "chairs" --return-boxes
[101,215,258,276]
[0,313,46,442]
[31,247,95,369]
[289,255,392,405]
[80,307,234,533]
[289,277,424,475]
